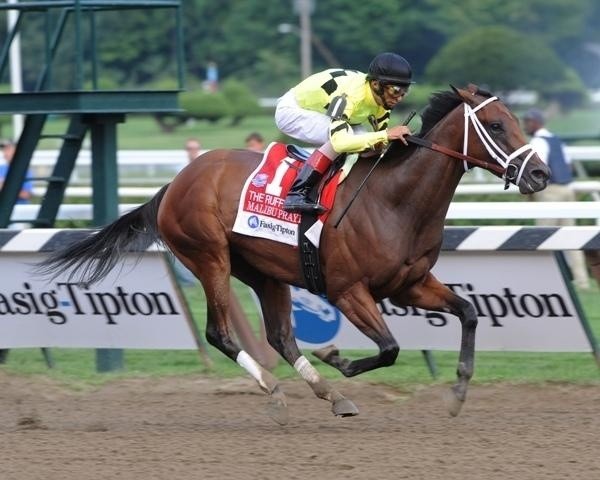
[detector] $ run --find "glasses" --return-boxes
[383,84,407,99]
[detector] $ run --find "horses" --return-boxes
[22,82,553,427]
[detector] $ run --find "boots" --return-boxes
[282,149,334,215]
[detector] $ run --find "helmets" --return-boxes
[365,52,417,84]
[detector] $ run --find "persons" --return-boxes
[246,133,265,151]
[0,140,34,204]
[177,138,201,171]
[274,51,413,213]
[521,109,591,292]
